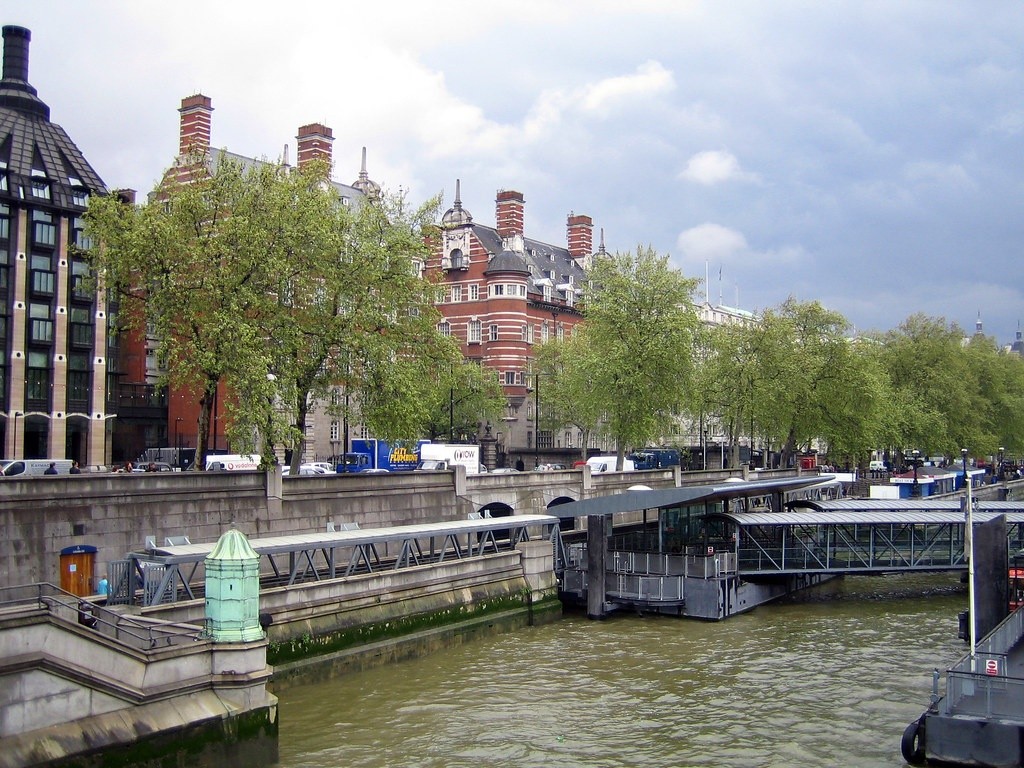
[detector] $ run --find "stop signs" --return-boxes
[988,661,996,668]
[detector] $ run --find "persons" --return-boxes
[899,463,911,474]
[823,463,842,472]
[516,455,524,471]
[136,575,144,588]
[0,465,4,476]
[112,467,119,473]
[44,462,58,474]
[98,574,112,595]
[70,462,81,474]
[145,463,158,472]
[932,461,947,469]
[690,460,695,470]
[122,462,133,473]
[749,460,756,470]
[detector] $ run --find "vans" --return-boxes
[303,462,334,470]
[134,463,173,472]
[870,461,887,471]
[327,452,372,472]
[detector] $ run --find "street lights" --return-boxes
[526,387,538,468]
[912,448,920,496]
[999,446,1005,480]
[961,447,968,488]
[13,412,23,459]
[175,417,184,472]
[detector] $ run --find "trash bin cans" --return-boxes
[78,602,96,629]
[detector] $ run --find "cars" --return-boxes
[361,469,390,473]
[491,468,519,474]
[542,464,567,470]
[282,466,336,475]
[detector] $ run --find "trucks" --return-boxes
[186,454,261,471]
[631,448,680,469]
[413,443,480,475]
[350,437,431,471]
[0,459,73,476]
[586,456,634,473]
